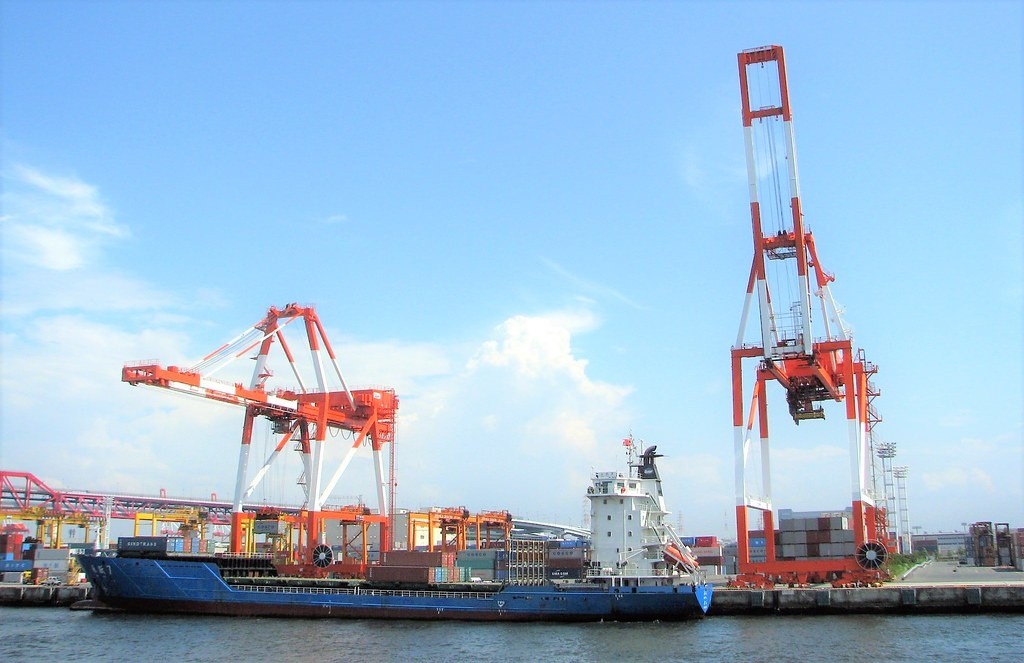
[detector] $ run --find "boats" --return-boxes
[67,425,716,620]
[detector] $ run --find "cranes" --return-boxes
[725,45,900,593]
[121,303,402,580]
